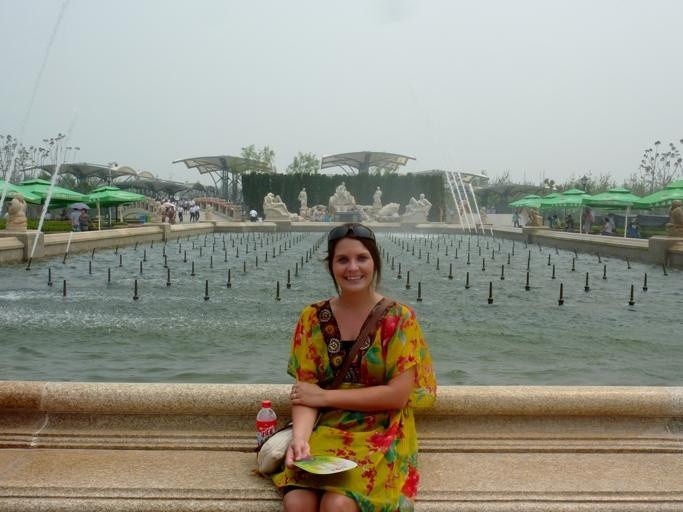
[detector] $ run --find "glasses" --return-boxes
[328,224,376,241]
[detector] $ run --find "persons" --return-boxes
[250,210,258,221]
[298,187,308,207]
[509,210,641,236]
[71,208,81,232]
[157,194,200,222]
[81,209,89,232]
[418,194,431,207]
[372,186,382,209]
[272,223,436,512]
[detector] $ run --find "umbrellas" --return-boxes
[70,203,90,209]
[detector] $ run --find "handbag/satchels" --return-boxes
[256,411,323,479]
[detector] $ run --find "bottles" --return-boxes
[255,399,279,454]
